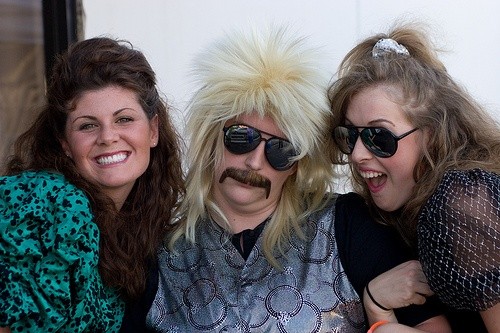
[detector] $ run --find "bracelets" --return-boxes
[365,282,390,312]
[367,320,389,333]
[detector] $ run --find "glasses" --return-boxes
[222,123,301,171]
[331,121,421,159]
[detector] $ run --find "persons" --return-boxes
[144,29,452,333]
[0,35,185,333]
[327,21,500,333]
